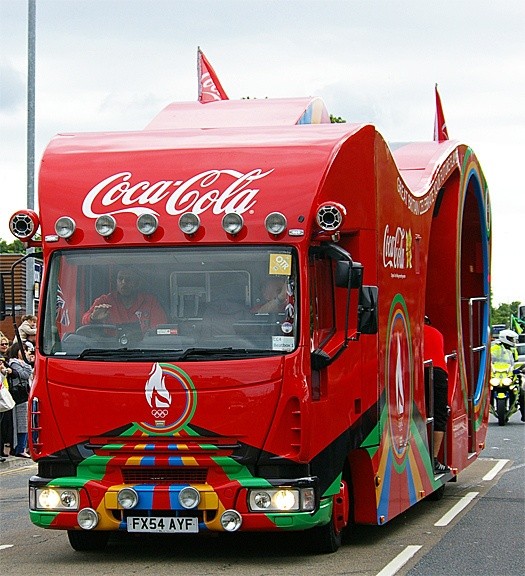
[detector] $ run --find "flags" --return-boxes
[432,85,449,139]
[198,52,230,102]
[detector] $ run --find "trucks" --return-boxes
[0,99,490,553]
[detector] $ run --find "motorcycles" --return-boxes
[491,344,518,427]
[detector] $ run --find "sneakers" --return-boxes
[433,462,450,474]
[15,452,31,458]
[0,455,5,462]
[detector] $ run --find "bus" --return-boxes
[492,324,506,337]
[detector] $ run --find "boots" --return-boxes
[519,394,524,423]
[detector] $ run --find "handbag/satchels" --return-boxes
[6,369,29,405]
[0,380,16,412]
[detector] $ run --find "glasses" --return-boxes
[0,343,9,346]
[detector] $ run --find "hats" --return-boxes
[424,314,430,324]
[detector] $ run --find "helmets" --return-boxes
[498,328,519,347]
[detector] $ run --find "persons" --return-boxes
[251,274,295,313]
[28,351,35,367]
[26,334,36,347]
[422,313,453,474]
[0,338,9,358]
[491,329,524,424]
[0,360,8,461]
[5,343,33,458]
[79,265,167,333]
[11,314,38,350]
